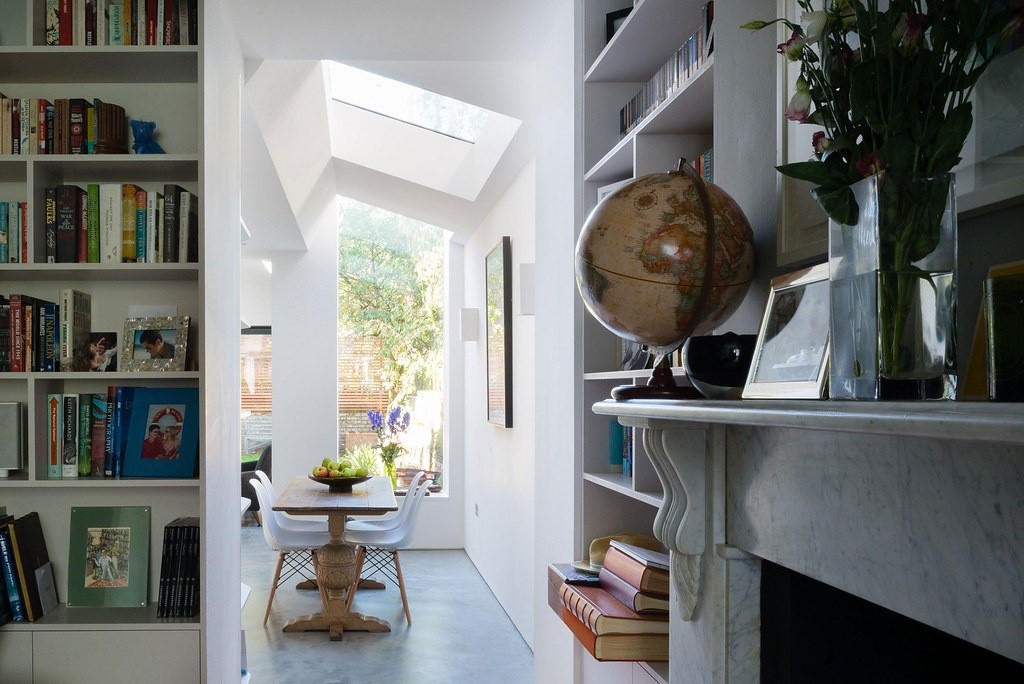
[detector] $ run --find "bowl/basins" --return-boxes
[684,335,759,399]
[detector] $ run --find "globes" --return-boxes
[573,157,760,401]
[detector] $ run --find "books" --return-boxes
[0,287,92,373]
[1,506,200,624]
[47,0,200,46]
[618,1,716,141]
[0,183,198,265]
[613,334,688,369]
[46,386,149,479]
[122,387,200,478]
[606,419,633,477]
[690,148,716,186]
[0,92,104,156]
[546,537,669,664]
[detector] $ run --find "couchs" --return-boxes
[241,443,273,526]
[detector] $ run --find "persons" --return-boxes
[92,548,116,582]
[139,330,175,359]
[88,336,118,372]
[142,423,181,459]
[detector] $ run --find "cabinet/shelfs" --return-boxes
[573,0,783,683]
[0,0,242,684]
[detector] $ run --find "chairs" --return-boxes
[255,470,330,602]
[249,478,330,626]
[342,479,433,624]
[343,471,426,607]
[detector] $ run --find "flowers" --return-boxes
[366,406,410,461]
[740,0,1024,227]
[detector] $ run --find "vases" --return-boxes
[828,169,958,400]
[384,461,398,490]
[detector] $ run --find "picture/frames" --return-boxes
[742,261,829,399]
[122,387,198,478]
[120,316,191,372]
[485,235,513,428]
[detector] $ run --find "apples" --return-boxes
[313,457,369,478]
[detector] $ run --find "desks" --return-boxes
[592,396,1024,684]
[272,476,397,641]
